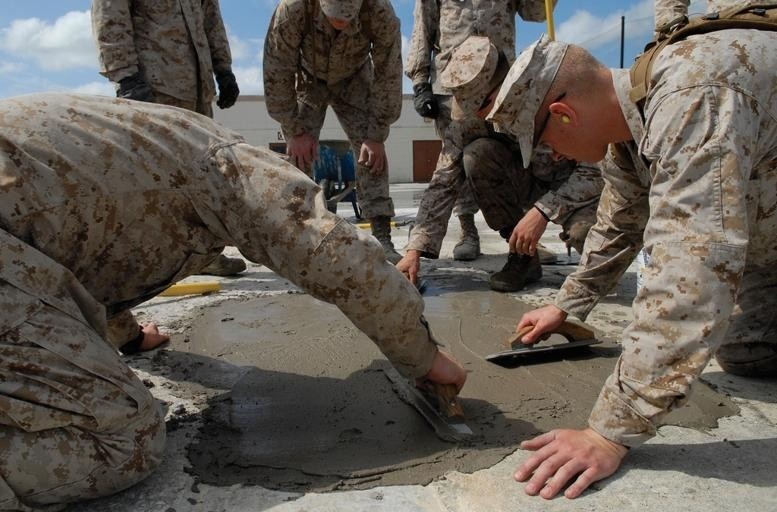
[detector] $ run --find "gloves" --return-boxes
[413,82,438,119]
[217,72,240,109]
[117,74,155,102]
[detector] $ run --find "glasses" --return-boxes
[533,113,552,154]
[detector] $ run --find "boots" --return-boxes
[370,215,402,265]
[198,254,246,276]
[453,215,479,260]
[490,249,542,291]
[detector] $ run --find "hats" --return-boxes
[320,1,361,19]
[485,34,569,169]
[440,36,498,121]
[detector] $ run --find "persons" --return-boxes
[655,0,746,36]
[0,93,466,512]
[263,0,403,265]
[395,36,605,292]
[405,1,557,264]
[91,1,246,275]
[486,0,777,499]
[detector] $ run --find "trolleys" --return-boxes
[310,139,361,220]
